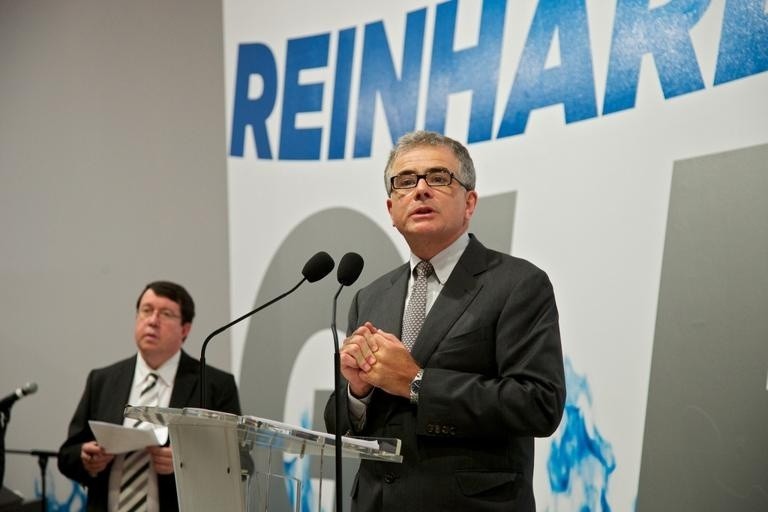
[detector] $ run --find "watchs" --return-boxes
[409,369,424,404]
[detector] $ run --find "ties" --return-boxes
[399,260,434,354]
[117,370,160,512]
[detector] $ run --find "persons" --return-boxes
[57,281,255,512]
[323,133,566,512]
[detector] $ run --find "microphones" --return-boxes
[0,382,38,412]
[332,252,364,354]
[200,251,335,409]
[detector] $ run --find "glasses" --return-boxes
[388,167,472,196]
[137,305,183,322]
